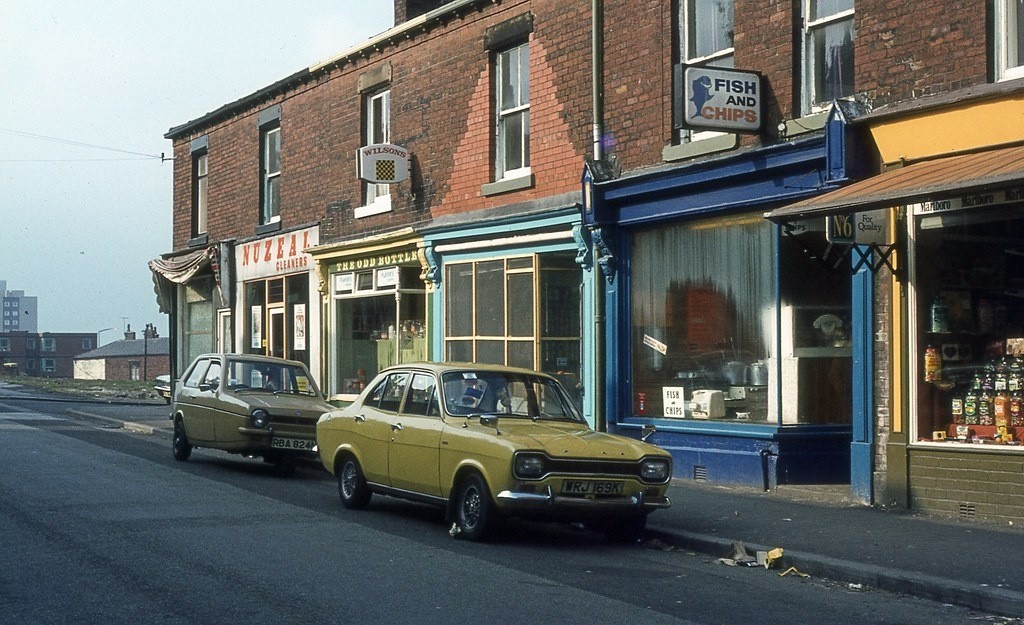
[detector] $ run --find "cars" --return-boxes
[167,354,343,478]
[317,360,674,546]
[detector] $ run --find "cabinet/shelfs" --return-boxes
[925,329,987,388]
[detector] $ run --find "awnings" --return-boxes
[147,246,225,312]
[761,145,1024,275]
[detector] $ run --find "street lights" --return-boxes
[121,316,129,334]
[98,326,120,348]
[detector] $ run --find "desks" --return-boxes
[949,424,1024,445]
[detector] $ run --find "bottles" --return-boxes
[948,357,1024,428]
[402,319,424,339]
[924,343,942,382]
[927,295,948,332]
